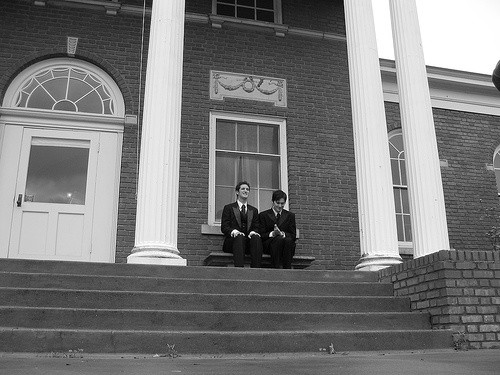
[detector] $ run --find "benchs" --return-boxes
[204,253,316,270]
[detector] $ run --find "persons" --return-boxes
[220,182,264,268]
[259,189,296,268]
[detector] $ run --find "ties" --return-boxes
[276,213,281,221]
[241,205,246,214]
